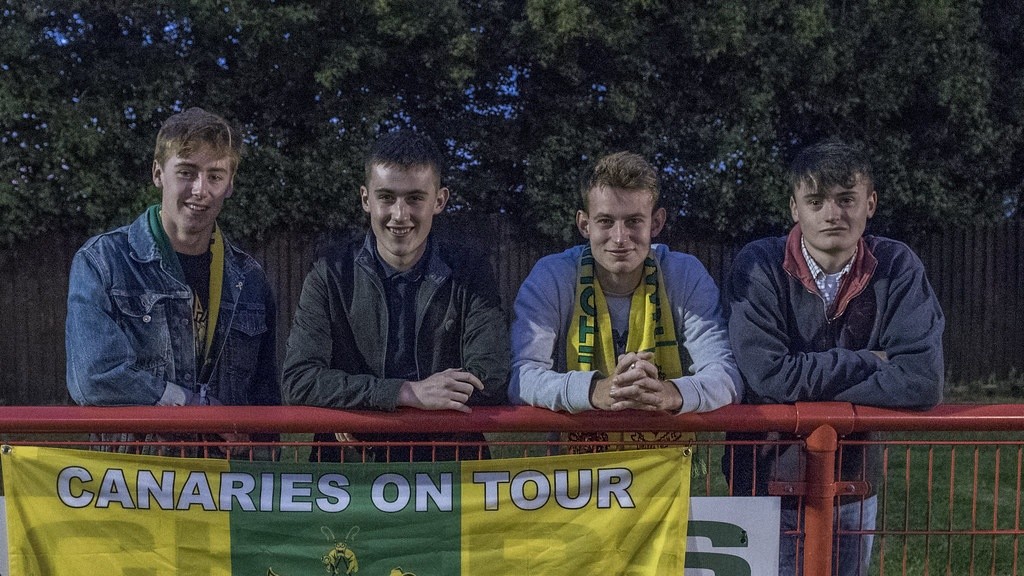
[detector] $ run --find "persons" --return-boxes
[66,108,281,461]
[510,151,744,457]
[282,130,510,460]
[722,143,946,576]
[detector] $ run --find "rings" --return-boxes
[631,361,636,369]
